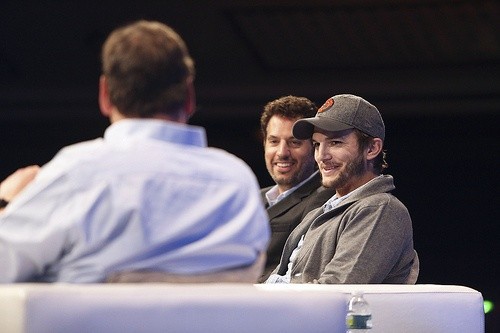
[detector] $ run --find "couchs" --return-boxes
[0,280,484,333]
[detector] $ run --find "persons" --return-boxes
[0,23,271,284]
[265,94,415,284]
[259,97,338,284]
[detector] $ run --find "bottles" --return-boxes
[345,290,372,333]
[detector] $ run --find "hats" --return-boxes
[292,94,385,139]
